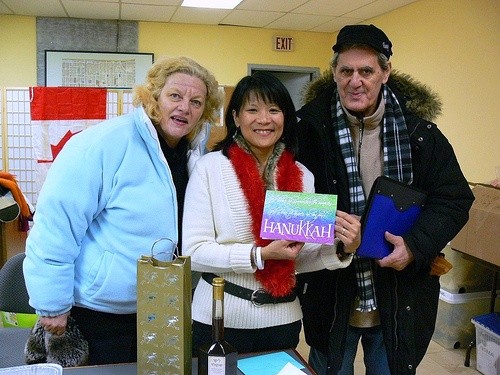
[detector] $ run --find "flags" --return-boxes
[29,87,107,195]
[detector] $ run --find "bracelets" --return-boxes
[257,247,264,270]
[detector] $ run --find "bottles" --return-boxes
[197,277,238,375]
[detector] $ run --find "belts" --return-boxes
[202,271,296,306]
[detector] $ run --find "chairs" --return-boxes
[0,248,62,368]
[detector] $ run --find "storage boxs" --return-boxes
[431,176,500,375]
[136,254,192,375]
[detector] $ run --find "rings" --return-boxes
[341,229,345,234]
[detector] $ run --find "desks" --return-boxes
[0,347,317,375]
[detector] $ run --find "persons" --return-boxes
[23,56,224,366]
[183,71,361,357]
[295,24,476,375]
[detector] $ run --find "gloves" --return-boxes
[25,318,89,366]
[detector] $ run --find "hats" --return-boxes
[332,24,393,60]
